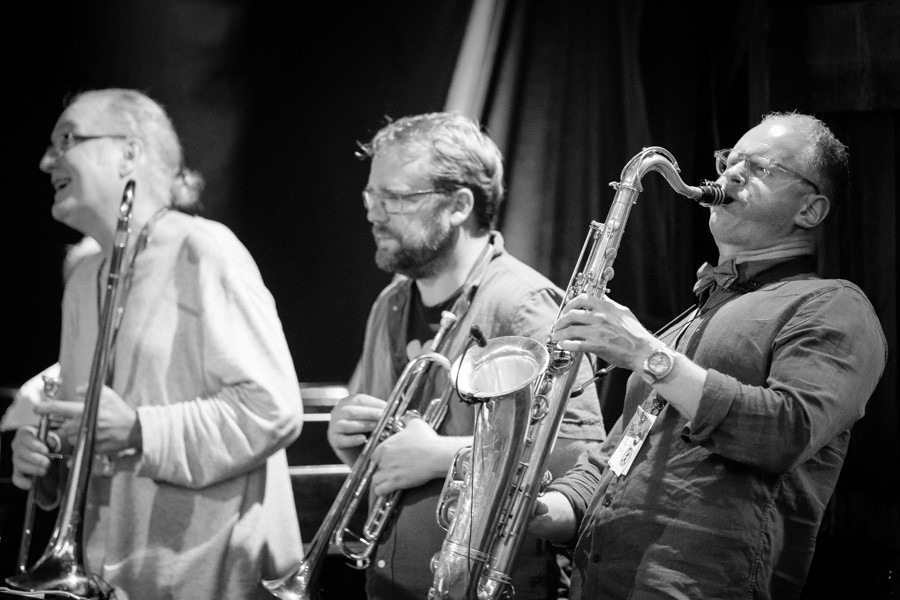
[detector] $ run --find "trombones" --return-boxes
[0,178,153,600]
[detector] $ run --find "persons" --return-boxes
[9,88,309,600]
[513,114,889,600]
[329,113,606,599]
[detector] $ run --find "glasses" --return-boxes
[48,129,127,158]
[362,189,447,214]
[714,148,822,196]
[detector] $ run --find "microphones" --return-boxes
[455,325,487,403]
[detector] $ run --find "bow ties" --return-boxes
[694,260,738,293]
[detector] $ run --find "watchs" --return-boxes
[639,343,680,385]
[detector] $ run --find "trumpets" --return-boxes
[262,307,458,600]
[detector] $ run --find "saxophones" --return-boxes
[426,143,730,600]
